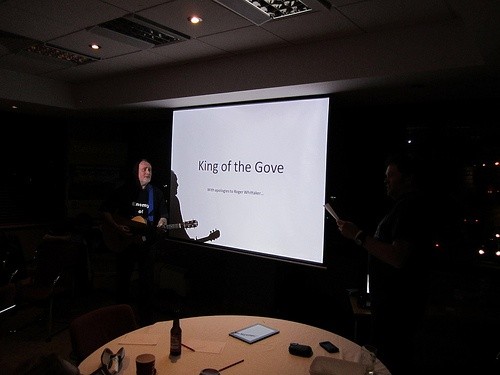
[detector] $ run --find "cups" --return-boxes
[135,353,157,375]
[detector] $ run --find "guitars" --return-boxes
[103,214,197,253]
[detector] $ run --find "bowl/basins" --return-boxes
[200,368,220,375]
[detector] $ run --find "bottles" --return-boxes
[170,319,182,357]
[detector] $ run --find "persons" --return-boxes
[338,153,437,374]
[98,158,168,320]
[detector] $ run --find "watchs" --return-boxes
[355,230,367,246]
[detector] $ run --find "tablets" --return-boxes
[229,323,279,344]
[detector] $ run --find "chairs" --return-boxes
[69,304,136,360]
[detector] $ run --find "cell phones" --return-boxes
[319,342,339,353]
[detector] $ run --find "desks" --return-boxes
[78,315,392,375]
